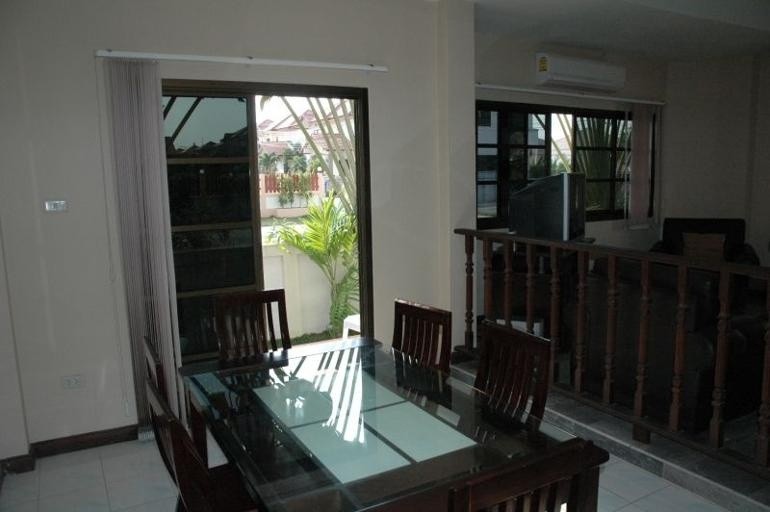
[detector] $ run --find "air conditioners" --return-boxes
[535,51,626,92]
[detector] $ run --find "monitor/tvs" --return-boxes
[506,172,586,255]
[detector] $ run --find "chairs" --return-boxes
[456,388,542,445]
[394,351,452,410]
[447,437,587,512]
[474,315,552,419]
[391,298,452,374]
[142,336,168,406]
[145,381,257,512]
[214,289,291,358]
[215,349,288,376]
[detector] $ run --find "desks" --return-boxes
[178,335,610,512]
